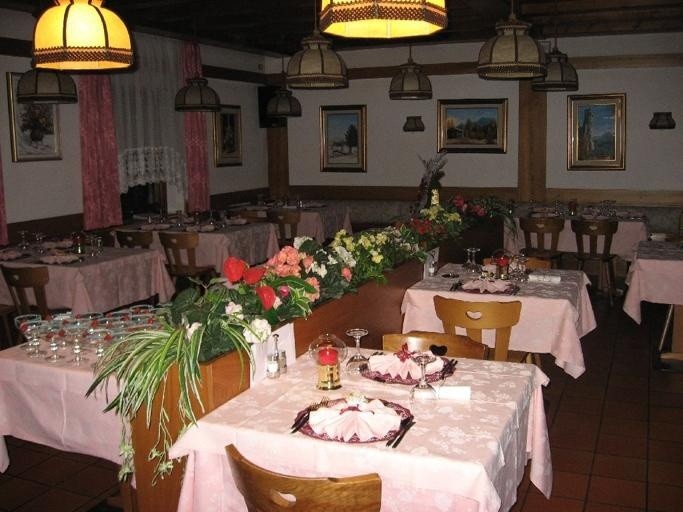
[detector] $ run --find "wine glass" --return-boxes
[462,248,473,270]
[17,231,30,249]
[345,328,371,375]
[207,210,216,223]
[34,233,47,255]
[14,305,169,367]
[410,355,437,403]
[467,248,481,272]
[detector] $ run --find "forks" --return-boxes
[291,396,330,433]
[290,401,318,428]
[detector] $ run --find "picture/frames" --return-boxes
[437,98,508,154]
[213,104,242,167]
[6,72,63,163]
[567,92,626,172]
[319,104,367,173]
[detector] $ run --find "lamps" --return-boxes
[31,0,134,70]
[389,46,432,100]
[266,55,302,117]
[16,61,78,105]
[648,112,675,129]
[174,46,221,112]
[286,0,348,90]
[530,38,578,92]
[319,0,448,39]
[476,0,548,81]
[403,116,424,132]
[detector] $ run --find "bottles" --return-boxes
[429,264,435,276]
[266,354,280,378]
[278,350,287,374]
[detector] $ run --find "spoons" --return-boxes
[386,416,413,446]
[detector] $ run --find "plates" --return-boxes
[295,398,412,442]
[361,351,455,384]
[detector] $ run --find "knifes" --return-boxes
[393,422,416,449]
